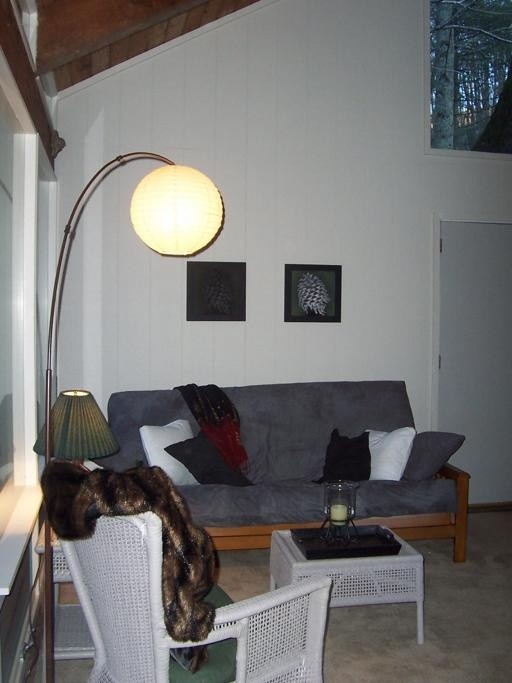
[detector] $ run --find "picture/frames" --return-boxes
[284,263,342,323]
[186,261,247,322]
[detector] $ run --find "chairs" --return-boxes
[61,511,333,683]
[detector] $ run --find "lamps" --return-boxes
[44,152,223,683]
[32,390,119,465]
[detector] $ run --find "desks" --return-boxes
[270,524,424,646]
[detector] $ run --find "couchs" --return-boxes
[108,379,471,563]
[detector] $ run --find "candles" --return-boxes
[330,504,348,526]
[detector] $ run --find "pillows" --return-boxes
[406,432,465,482]
[313,428,370,485]
[164,432,254,486]
[359,425,417,482]
[139,419,200,486]
[94,414,145,474]
